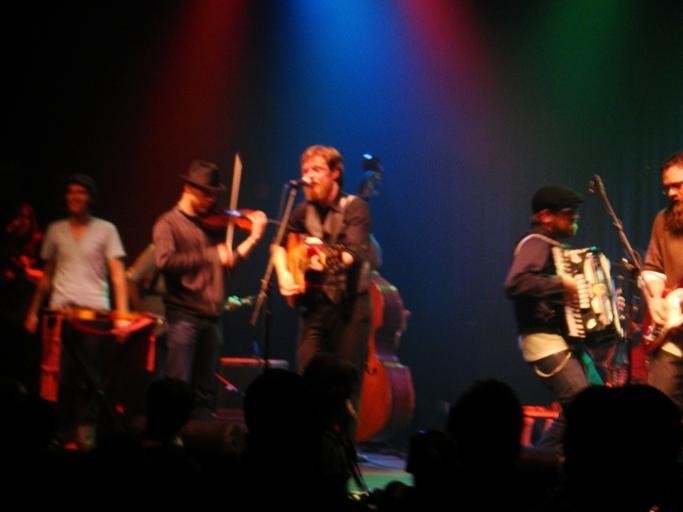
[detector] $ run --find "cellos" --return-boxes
[356,154,414,443]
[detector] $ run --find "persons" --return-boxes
[500,382,625,455]
[0,364,228,512]
[24,174,131,336]
[0,200,47,400]
[625,384,683,512]
[281,144,372,451]
[150,160,268,412]
[636,152,682,406]
[125,242,167,323]
[499,455,628,512]
[228,356,501,512]
[501,185,625,455]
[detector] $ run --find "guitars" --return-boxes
[592,171,683,361]
[284,232,346,309]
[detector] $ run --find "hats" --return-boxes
[178,163,227,192]
[68,173,92,194]
[533,186,583,212]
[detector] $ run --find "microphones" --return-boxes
[289,178,313,189]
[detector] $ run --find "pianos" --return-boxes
[550,246,625,342]
[38,309,165,329]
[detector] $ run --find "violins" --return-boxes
[202,205,305,233]
